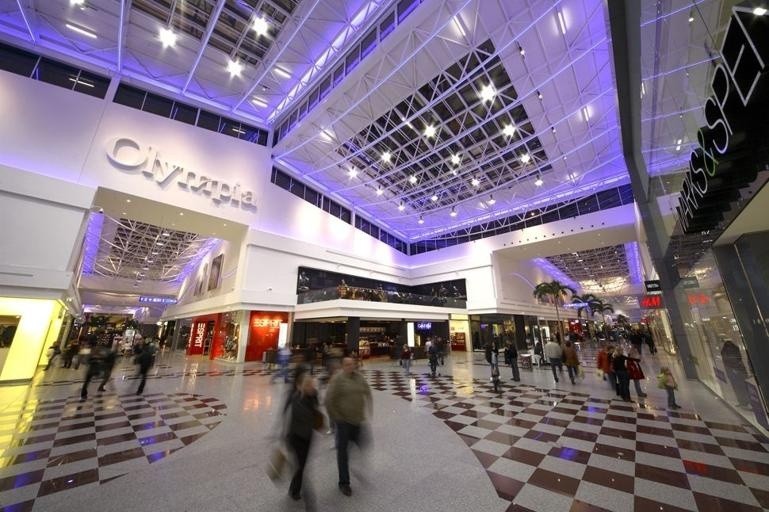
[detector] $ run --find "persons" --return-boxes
[657,367,681,409]
[337,278,461,307]
[485,341,499,381]
[392,334,445,375]
[270,340,375,501]
[526,330,580,384]
[597,323,655,401]
[43,333,153,402]
[506,339,520,381]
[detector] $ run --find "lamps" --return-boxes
[518,46,526,56]
[533,175,544,187]
[551,126,556,134]
[376,184,384,196]
[348,165,357,180]
[486,194,496,206]
[397,201,405,211]
[449,206,458,217]
[536,90,543,100]
[418,216,424,226]
